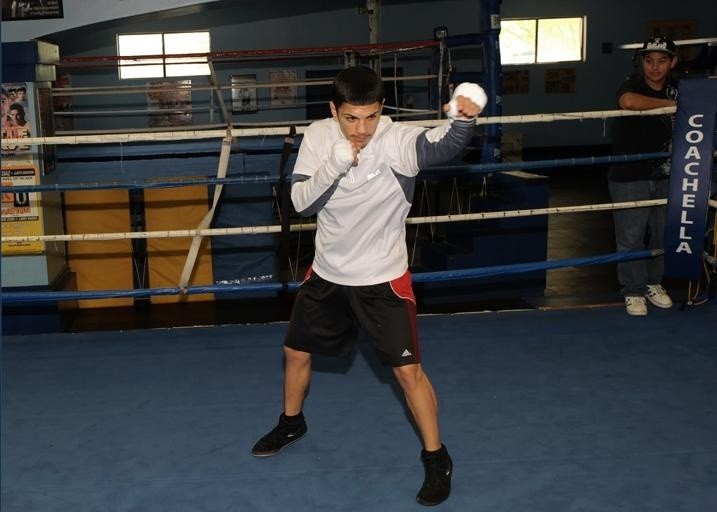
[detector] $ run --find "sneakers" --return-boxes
[415,442,454,507]
[624,295,648,316]
[251,410,309,458]
[644,283,673,309]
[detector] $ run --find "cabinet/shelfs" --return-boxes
[61,174,215,309]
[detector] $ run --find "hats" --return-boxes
[639,36,677,56]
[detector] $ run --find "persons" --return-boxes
[606,34,680,317]
[249,65,488,505]
[0,87,30,138]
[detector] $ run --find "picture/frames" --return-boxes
[229,74,258,115]
[269,70,298,108]
